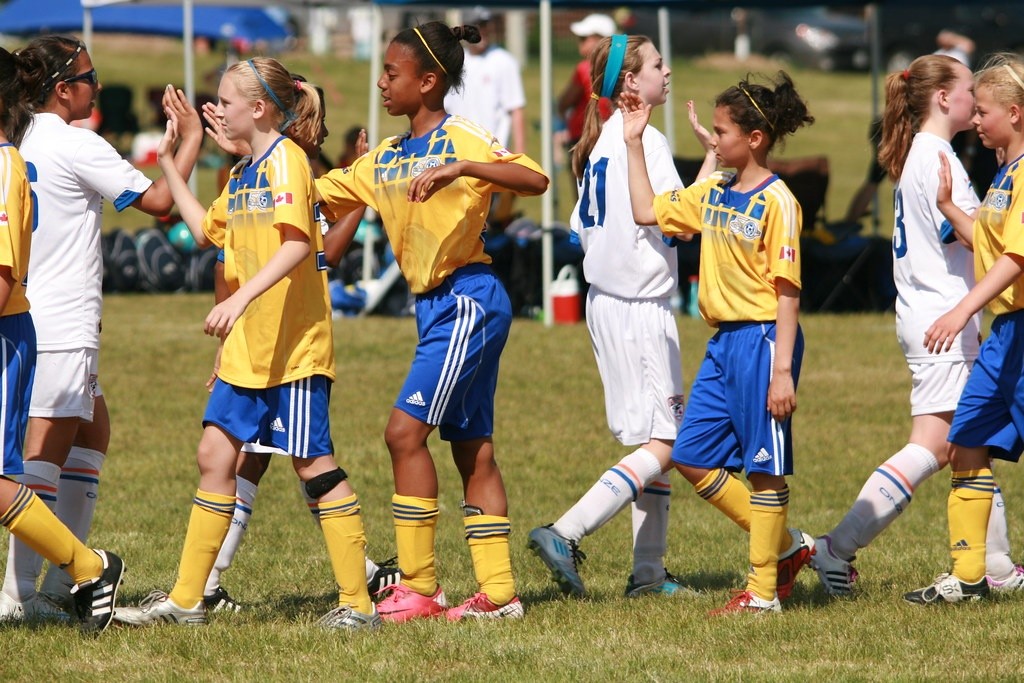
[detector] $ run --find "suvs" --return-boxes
[624,0,869,74]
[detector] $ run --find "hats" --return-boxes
[569,13,617,39]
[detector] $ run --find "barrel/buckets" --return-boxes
[551,264,582,324]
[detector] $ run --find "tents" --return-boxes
[1,0,290,51]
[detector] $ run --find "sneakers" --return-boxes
[368,556,402,599]
[203,586,249,614]
[986,564,1024,591]
[374,582,450,625]
[69,549,128,641]
[313,601,383,633]
[776,528,817,602]
[0,590,70,623]
[525,522,588,601]
[807,534,858,601]
[706,590,782,617]
[433,592,525,624]
[112,590,210,628]
[625,567,700,599]
[902,572,996,608]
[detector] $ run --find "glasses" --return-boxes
[46,68,97,93]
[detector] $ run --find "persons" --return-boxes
[807,54,1024,597]
[901,54,1024,608]
[845,12,1001,223]
[557,13,618,204]
[442,4,527,300]
[203,73,400,611]
[334,125,369,168]
[0,46,127,639]
[112,57,387,633]
[0,35,204,624]
[526,33,718,600]
[616,69,817,617]
[203,19,552,621]
